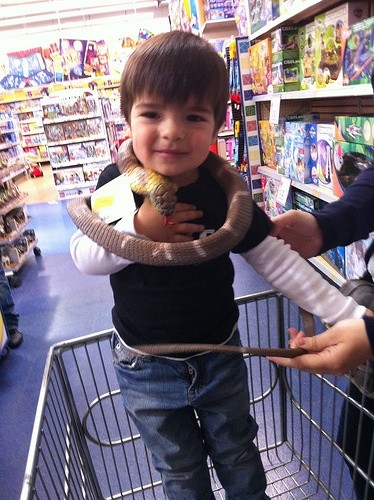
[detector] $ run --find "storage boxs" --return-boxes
[216,138,227,160]
[258,112,374,198]
[53,167,85,186]
[260,174,374,283]
[204,0,238,21]
[247,2,374,95]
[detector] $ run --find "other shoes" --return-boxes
[7,329,23,349]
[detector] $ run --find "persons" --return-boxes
[0,263,24,348]
[70,30,374,500]
[268,162,374,500]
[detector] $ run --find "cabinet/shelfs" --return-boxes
[249,0,374,287]
[0,18,237,289]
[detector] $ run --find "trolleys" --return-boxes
[20,285,374,500]
